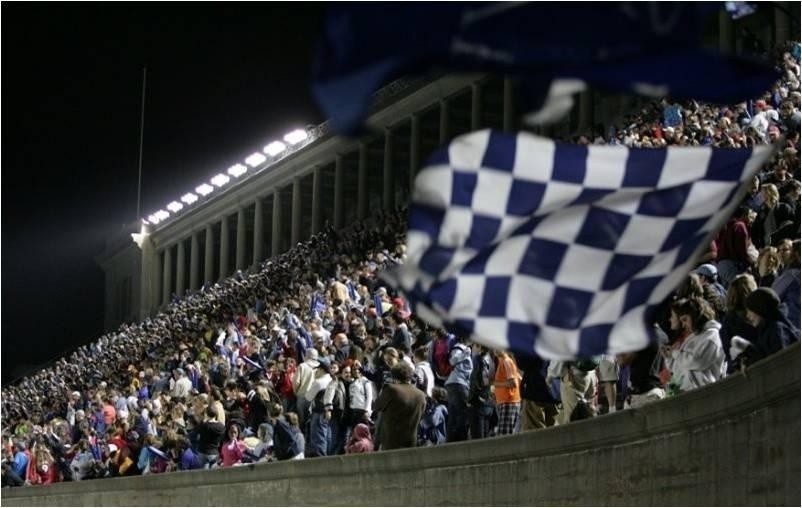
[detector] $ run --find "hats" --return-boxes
[691,263,718,280]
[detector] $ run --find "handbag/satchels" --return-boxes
[312,388,344,417]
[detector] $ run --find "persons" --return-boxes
[556,39,800,263]
[2,189,475,485]
[468,264,800,439]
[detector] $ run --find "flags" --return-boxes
[374,129,780,360]
[309,3,782,136]
